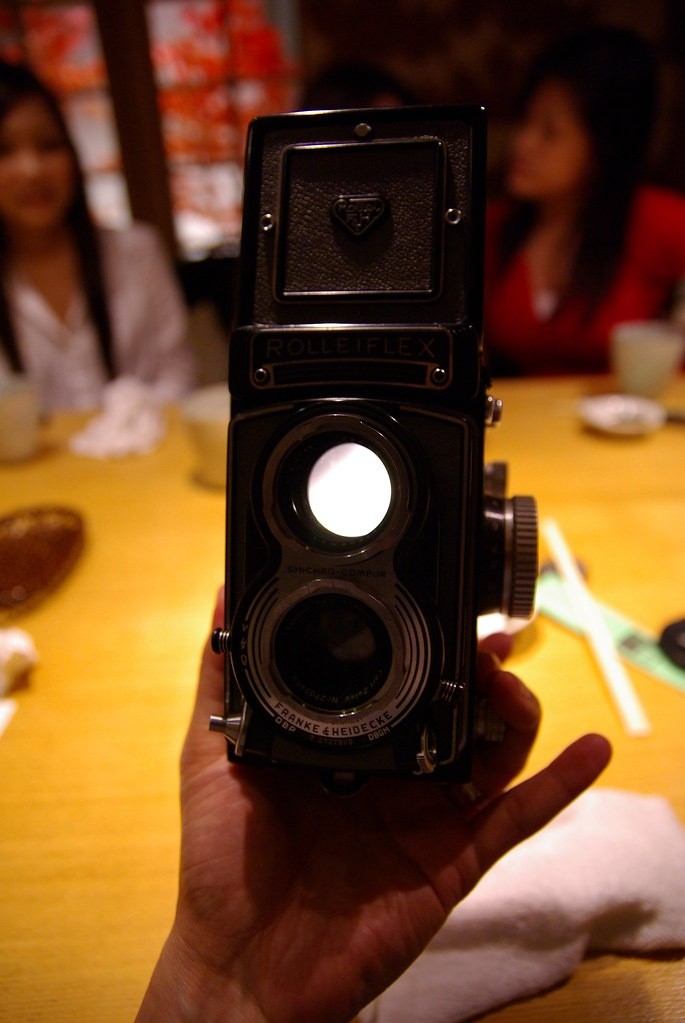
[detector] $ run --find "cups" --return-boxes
[190,383,232,484]
[0,377,39,456]
[610,326,681,398]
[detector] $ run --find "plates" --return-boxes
[574,392,667,436]
[0,500,84,620]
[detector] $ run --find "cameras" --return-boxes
[192,95,540,783]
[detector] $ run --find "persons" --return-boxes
[478,23,685,375]
[0,65,186,408]
[133,587,611,1022]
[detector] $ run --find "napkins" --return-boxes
[341,784,685,1023]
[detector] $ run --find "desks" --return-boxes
[0,362,685,1021]
